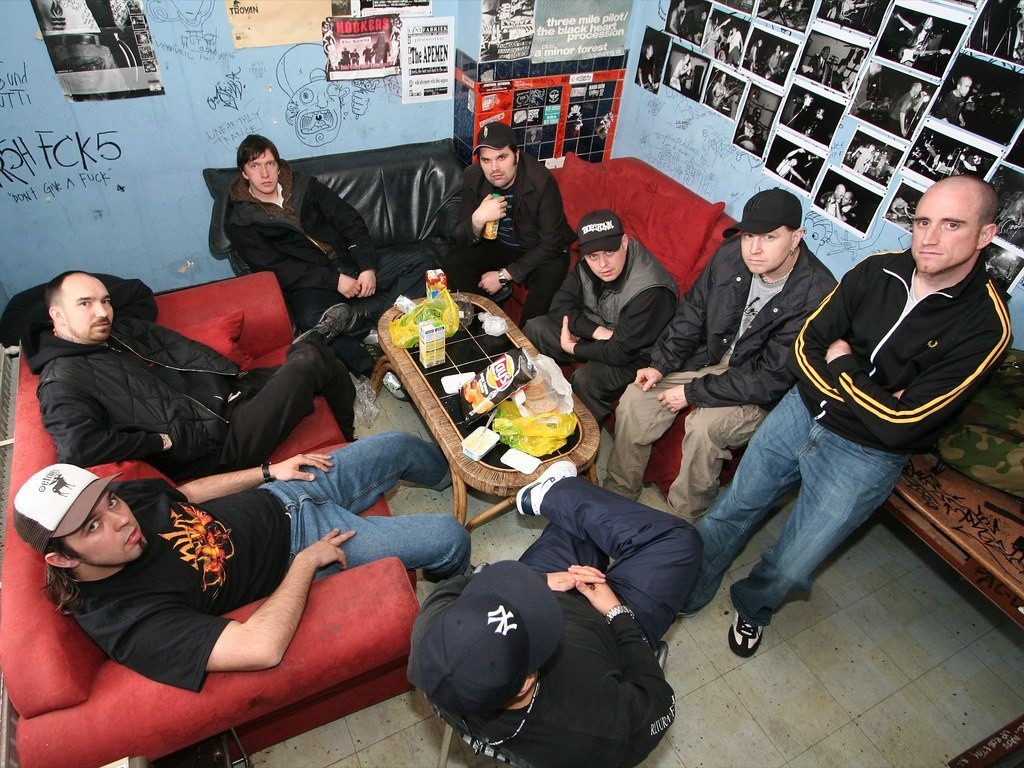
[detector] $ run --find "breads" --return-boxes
[523,376,559,416]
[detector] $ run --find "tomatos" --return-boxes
[471,380,479,389]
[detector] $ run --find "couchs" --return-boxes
[502,157,748,500]
[0,272,419,768]
[202,137,467,337]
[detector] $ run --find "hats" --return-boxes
[420,561,562,716]
[473,122,517,155]
[722,187,802,237]
[14,464,123,554]
[578,209,624,254]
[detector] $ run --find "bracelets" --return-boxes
[260,462,274,482]
[607,605,634,625]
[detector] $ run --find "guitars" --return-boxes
[842,0,875,17]
[896,44,952,64]
[702,18,731,50]
[711,86,739,109]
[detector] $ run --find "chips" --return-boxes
[465,389,495,413]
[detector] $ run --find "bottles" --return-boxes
[482,194,500,239]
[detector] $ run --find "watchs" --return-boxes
[499,269,508,285]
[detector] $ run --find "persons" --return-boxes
[679,176,1013,657]
[12,431,473,693]
[406,462,703,768]
[23,271,353,473]
[446,122,578,328]
[603,187,835,522]
[636,0,1024,226]
[227,135,438,378]
[523,209,678,425]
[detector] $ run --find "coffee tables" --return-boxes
[368,293,600,534]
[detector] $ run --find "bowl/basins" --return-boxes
[461,426,500,461]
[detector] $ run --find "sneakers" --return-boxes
[293,303,352,347]
[516,461,577,515]
[729,611,763,657]
[382,372,410,401]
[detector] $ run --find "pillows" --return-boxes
[557,152,725,286]
[174,311,251,371]
